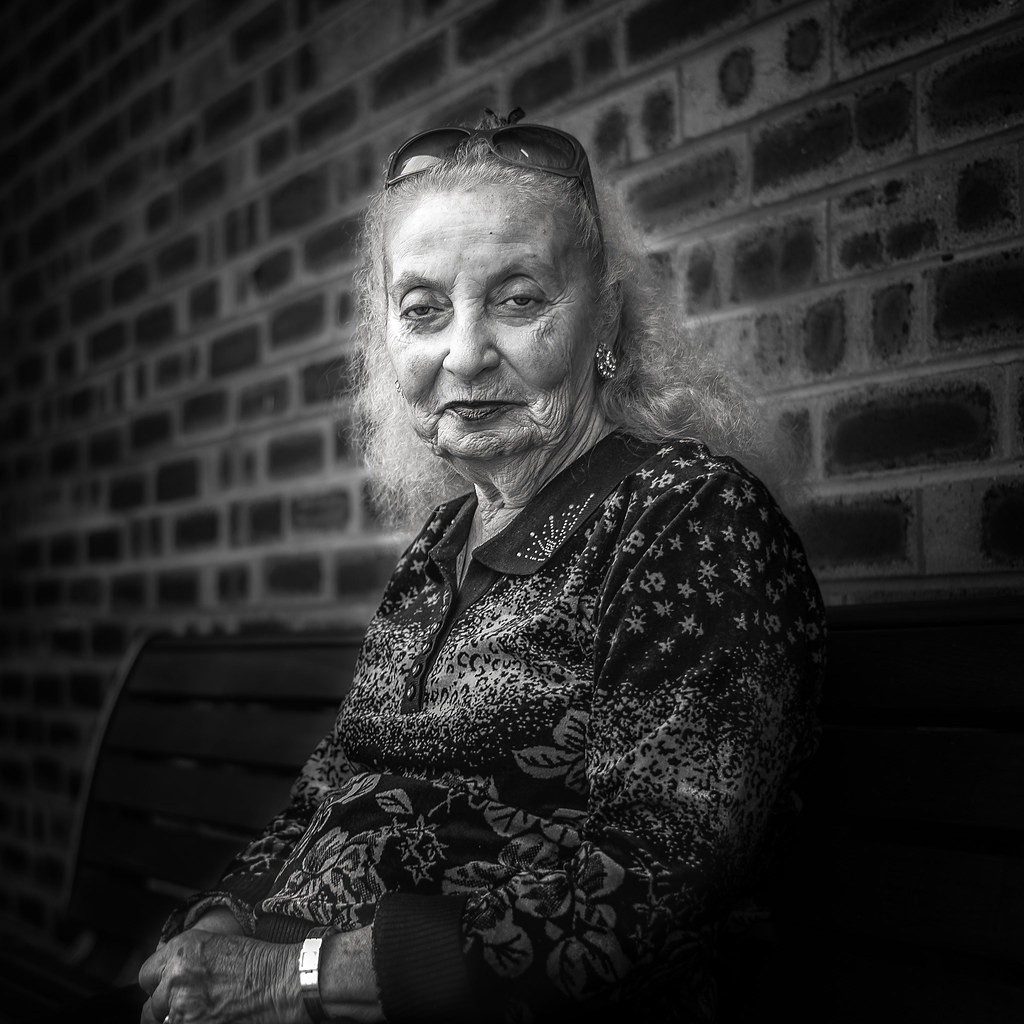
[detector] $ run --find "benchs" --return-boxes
[3,620,1024,1020]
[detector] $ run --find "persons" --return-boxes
[81,108,833,1024]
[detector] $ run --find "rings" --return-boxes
[163,1015,169,1022]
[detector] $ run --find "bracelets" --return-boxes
[298,927,339,1023]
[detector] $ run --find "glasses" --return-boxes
[384,123,608,255]
